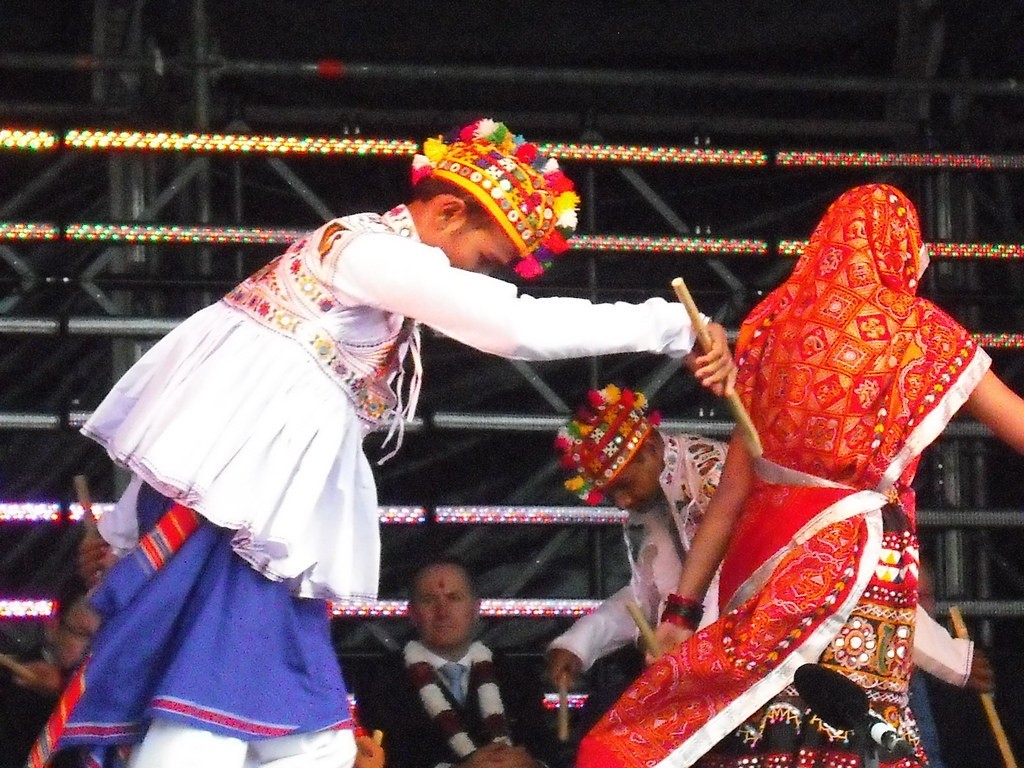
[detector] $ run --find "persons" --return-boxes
[357,558,552,768]
[29,118,737,768]
[578,183,1024,768]
[0,575,102,768]
[541,384,994,768]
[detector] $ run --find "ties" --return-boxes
[440,663,465,708]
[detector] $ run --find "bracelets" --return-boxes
[661,594,705,633]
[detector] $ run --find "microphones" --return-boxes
[794,663,915,756]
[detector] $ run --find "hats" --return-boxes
[411,116,581,280]
[554,384,660,504]
